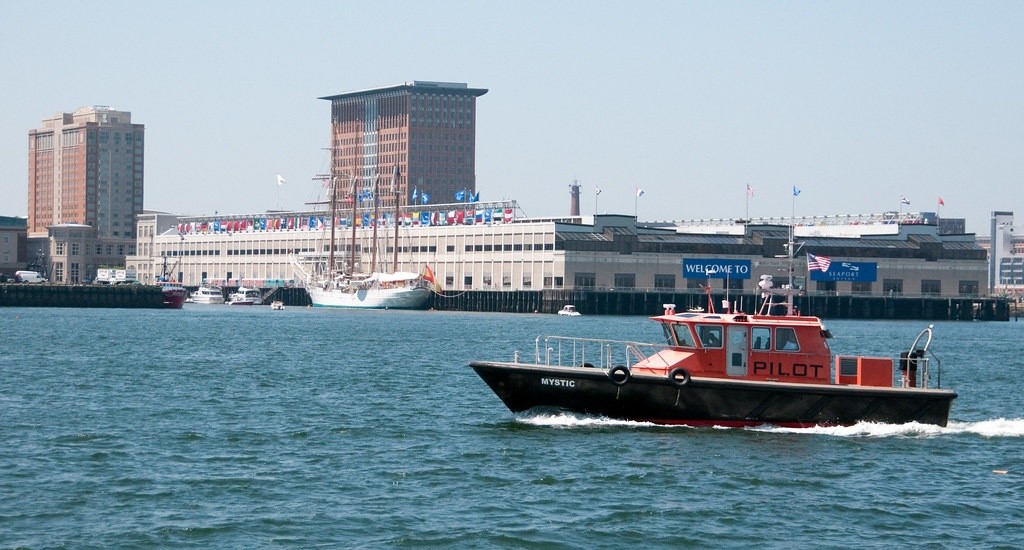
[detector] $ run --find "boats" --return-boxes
[271,302,284,310]
[228,287,263,305]
[154,257,188,309]
[190,280,225,305]
[558,305,581,317]
[469,229,956,429]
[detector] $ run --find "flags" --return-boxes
[594,185,602,196]
[792,185,801,196]
[635,186,645,198]
[899,195,910,205]
[938,196,945,206]
[423,265,443,293]
[178,187,517,236]
[746,185,754,197]
[278,175,288,186]
[806,251,831,273]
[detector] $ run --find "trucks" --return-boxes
[19,274,46,284]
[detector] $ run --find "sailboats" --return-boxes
[289,113,431,309]
